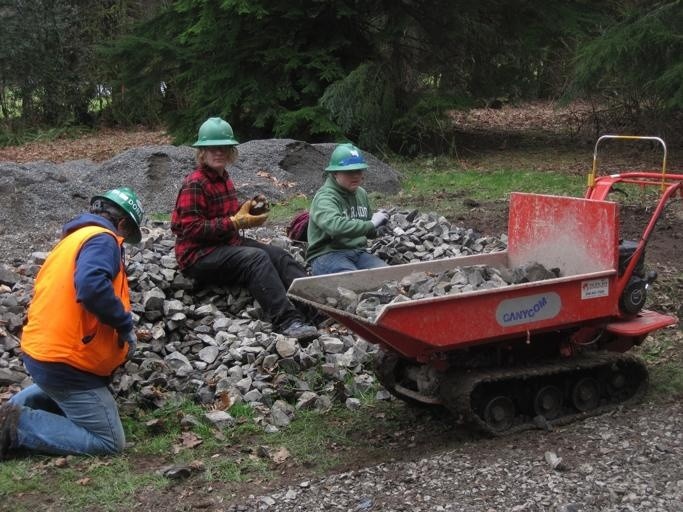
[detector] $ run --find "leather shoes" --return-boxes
[0,403,21,461]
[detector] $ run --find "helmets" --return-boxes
[90,187,145,245]
[324,143,369,172]
[193,116,240,147]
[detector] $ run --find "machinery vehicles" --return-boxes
[281,132,683,439]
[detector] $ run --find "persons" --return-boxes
[306,143,393,274]
[1,184,142,459]
[171,118,320,339]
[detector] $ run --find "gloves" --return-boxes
[230,200,269,232]
[371,210,391,229]
[118,328,138,361]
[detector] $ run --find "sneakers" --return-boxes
[283,321,319,340]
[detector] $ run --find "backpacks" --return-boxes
[287,212,308,242]
